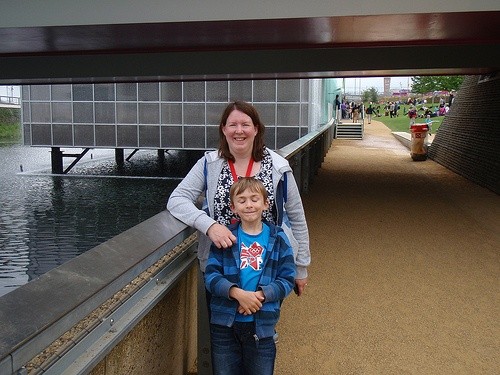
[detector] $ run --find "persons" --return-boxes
[166,102,311,375]
[206,178,297,375]
[333,91,456,136]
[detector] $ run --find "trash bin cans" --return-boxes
[409,124,429,161]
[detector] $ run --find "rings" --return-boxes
[305,283,309,288]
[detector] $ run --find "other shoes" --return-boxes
[273,329,280,343]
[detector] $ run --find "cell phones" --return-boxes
[294,285,299,294]
